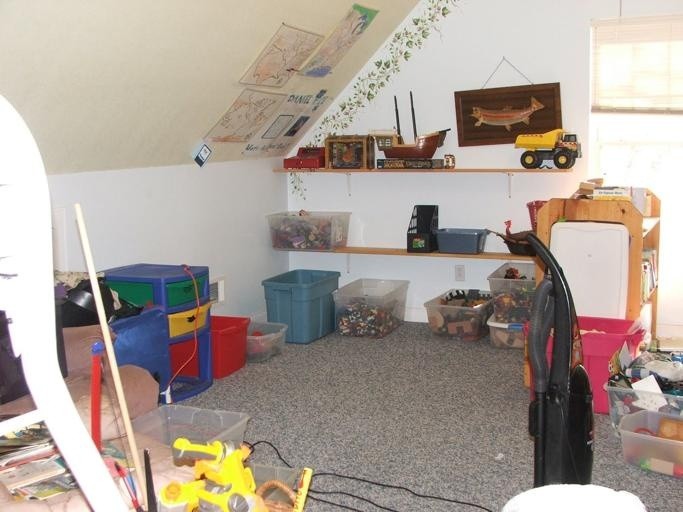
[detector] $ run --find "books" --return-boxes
[593,184,658,306]
[0,422,130,500]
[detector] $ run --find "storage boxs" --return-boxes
[323,135,375,170]
[330,276,409,338]
[484,312,523,350]
[263,208,352,251]
[432,226,491,254]
[421,287,497,342]
[243,321,288,363]
[261,267,342,345]
[239,462,297,505]
[520,310,682,481]
[486,262,538,324]
[208,314,251,379]
[129,403,249,455]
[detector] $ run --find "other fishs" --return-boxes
[469,96,545,132]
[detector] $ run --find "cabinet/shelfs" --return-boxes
[96,261,217,405]
[523,182,662,386]
[270,165,574,262]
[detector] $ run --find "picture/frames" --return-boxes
[453,81,561,147]
